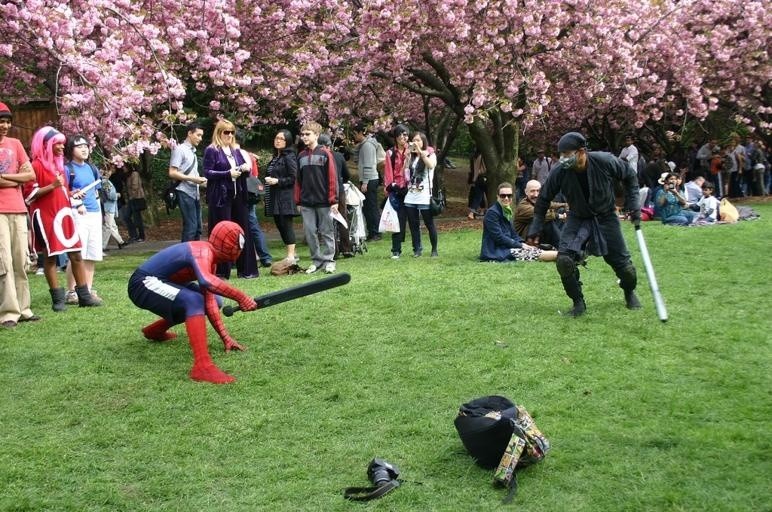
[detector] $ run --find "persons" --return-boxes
[0,102,41,326]
[619,135,771,229]
[169,123,207,243]
[320,118,457,263]
[467,145,569,219]
[294,121,340,272]
[478,182,560,261]
[235,145,272,267]
[527,132,641,316]
[202,119,259,278]
[24,125,145,308]
[265,129,299,264]
[128,219,255,384]
[514,178,571,249]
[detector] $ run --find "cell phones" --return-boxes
[669,182,674,189]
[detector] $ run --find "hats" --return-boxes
[0,102,12,118]
[557,132,586,152]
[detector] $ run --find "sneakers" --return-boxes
[624,292,640,309]
[263,252,438,274]
[3,284,102,326]
[566,302,586,317]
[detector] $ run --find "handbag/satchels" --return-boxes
[376,142,385,170]
[477,175,487,192]
[455,395,549,489]
[245,176,265,205]
[134,199,145,211]
[163,183,179,211]
[429,186,446,216]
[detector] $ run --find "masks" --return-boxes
[559,156,576,171]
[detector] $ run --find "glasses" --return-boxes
[223,130,236,135]
[498,194,514,198]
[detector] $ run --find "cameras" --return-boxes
[367,457,399,486]
[408,142,415,152]
